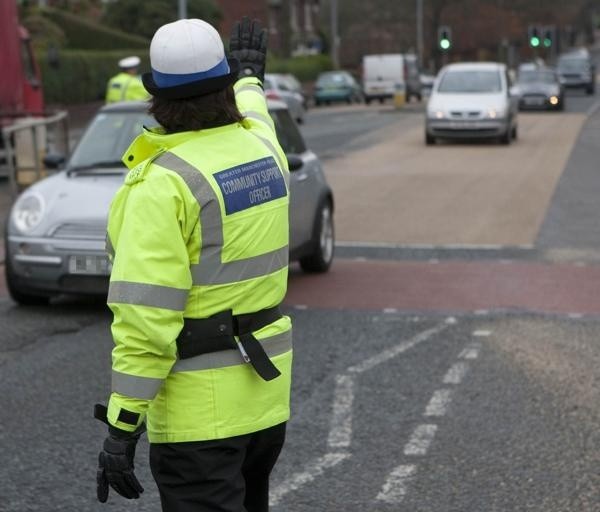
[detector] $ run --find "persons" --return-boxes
[104,54,151,102]
[94,14,295,510]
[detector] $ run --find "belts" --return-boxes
[177,305,287,341]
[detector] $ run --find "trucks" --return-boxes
[0,0,46,129]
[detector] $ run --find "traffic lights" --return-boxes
[541,23,555,51]
[437,24,453,52]
[526,24,541,49]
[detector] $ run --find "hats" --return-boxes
[116,56,141,69]
[141,18,240,100]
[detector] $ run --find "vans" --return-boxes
[360,52,425,104]
[425,61,519,144]
[558,47,596,95]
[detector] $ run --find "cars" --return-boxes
[508,65,566,111]
[5,92,338,308]
[263,72,309,125]
[314,69,364,106]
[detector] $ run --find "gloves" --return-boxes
[94,419,145,503]
[225,15,268,83]
